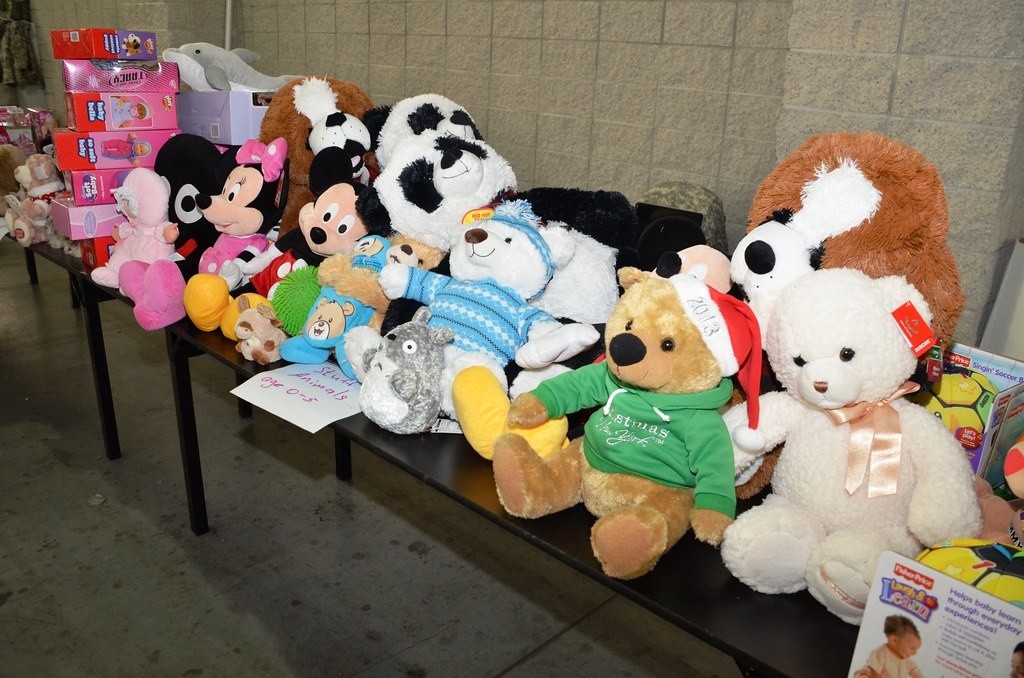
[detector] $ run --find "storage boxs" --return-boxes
[63,172,154,207]
[176,89,276,147]
[53,128,181,172]
[51,198,129,240]
[63,58,183,93]
[80,239,117,269]
[64,93,178,133]
[49,27,158,59]
[0,105,52,151]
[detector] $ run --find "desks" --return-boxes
[10,235,863,678]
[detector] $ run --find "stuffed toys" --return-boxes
[0,135,81,258]
[91,75,1024,625]
[163,42,307,92]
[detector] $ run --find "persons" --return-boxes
[101,133,152,166]
[1010,641,1024,678]
[853,615,924,678]
